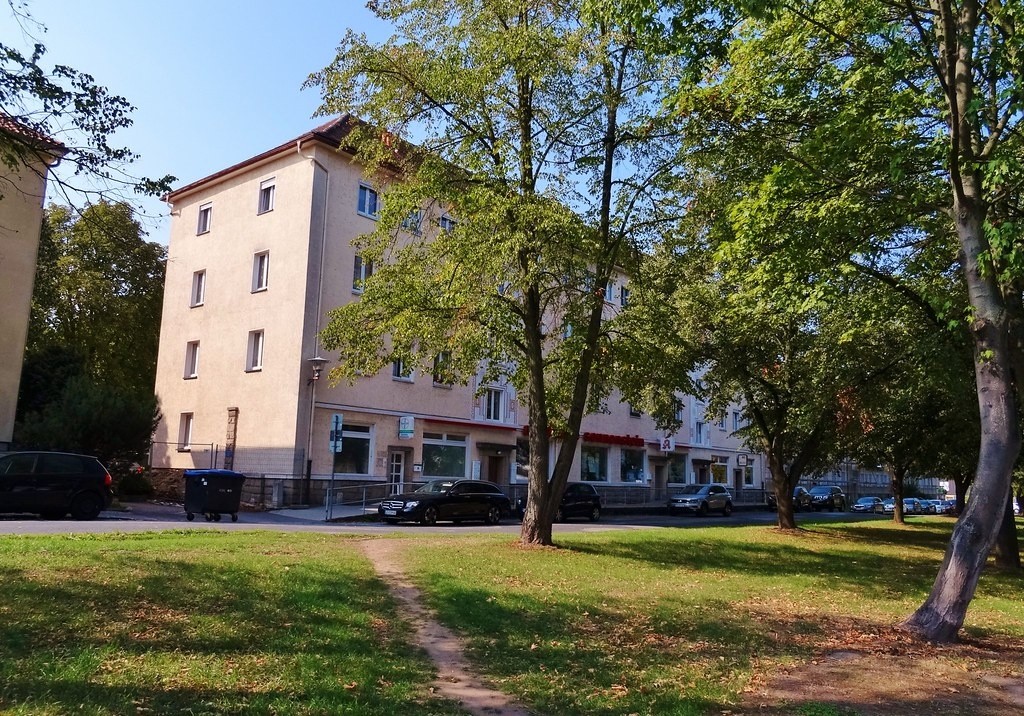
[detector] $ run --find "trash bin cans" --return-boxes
[182,467,247,523]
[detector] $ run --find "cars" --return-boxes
[850,497,884,514]
[941,500,948,513]
[945,500,957,517]
[669,484,733,516]
[768,486,812,512]
[930,500,941,514]
[378,478,512,526]
[919,500,937,515]
[516,481,601,523]
[903,498,923,515]
[0,451,114,520]
[884,497,908,514]
[808,486,845,511]
